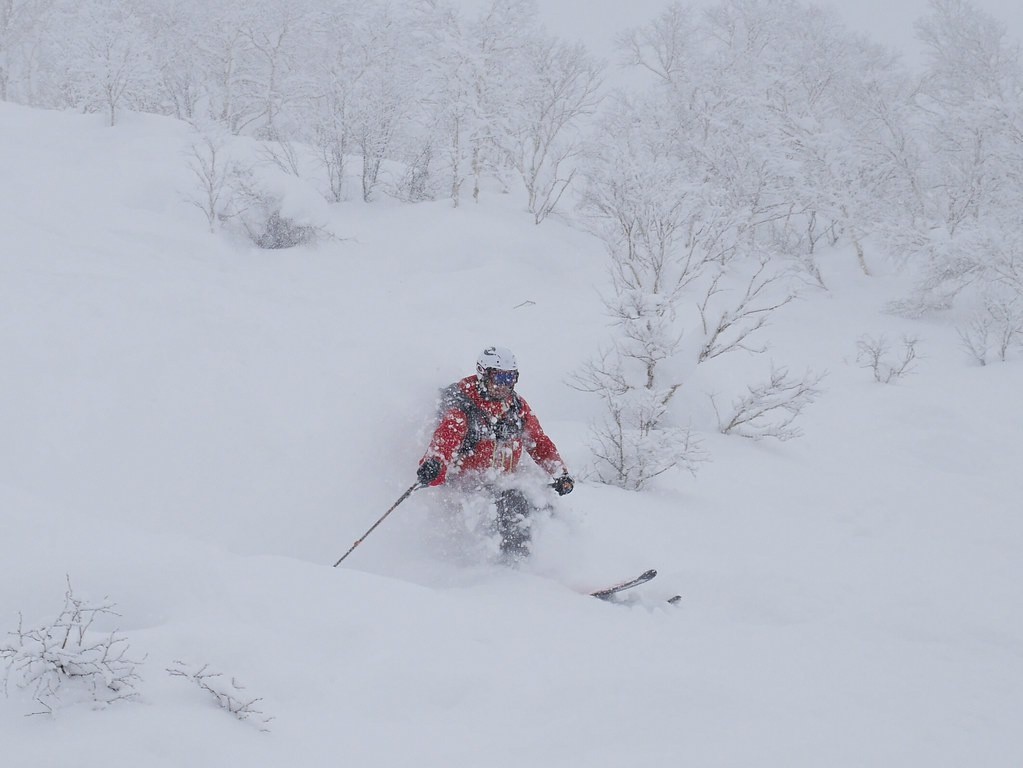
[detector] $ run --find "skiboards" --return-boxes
[585,567,683,605]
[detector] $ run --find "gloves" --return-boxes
[552,476,573,495]
[417,460,440,485]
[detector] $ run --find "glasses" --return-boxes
[490,372,516,386]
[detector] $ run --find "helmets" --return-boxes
[475,347,517,382]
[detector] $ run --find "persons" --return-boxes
[418,346,574,566]
